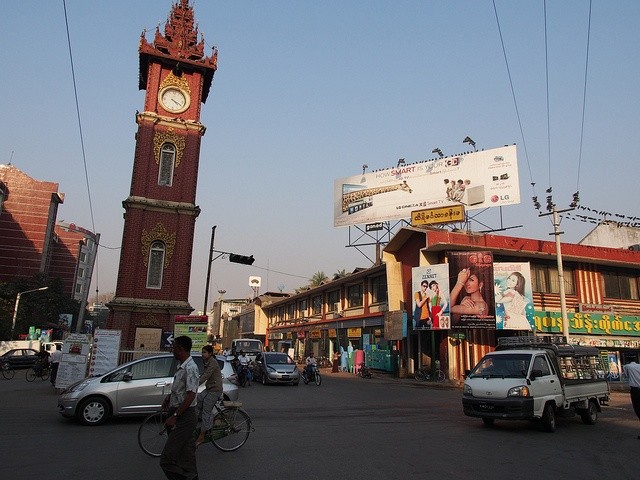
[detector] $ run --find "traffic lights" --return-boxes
[229,253,255,265]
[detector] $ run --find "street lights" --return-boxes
[10,287,48,340]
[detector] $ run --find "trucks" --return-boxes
[462,336,611,432]
[228,339,263,364]
[0,340,64,368]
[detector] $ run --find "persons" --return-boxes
[450,267,489,322]
[306,351,318,382]
[34,345,48,376]
[414,280,431,329]
[623,354,639,422]
[159,335,199,479]
[494,272,532,330]
[236,349,254,387]
[49,342,64,382]
[332,351,341,372]
[194,345,223,448]
[429,282,447,330]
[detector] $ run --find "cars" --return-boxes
[0,349,40,370]
[56,353,239,425]
[252,352,300,385]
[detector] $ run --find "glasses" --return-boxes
[423,284,428,288]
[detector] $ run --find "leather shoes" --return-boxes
[196,438,206,446]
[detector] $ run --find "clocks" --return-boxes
[158,84,191,113]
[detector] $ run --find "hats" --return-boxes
[430,280,439,289]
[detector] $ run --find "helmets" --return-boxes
[309,351,315,356]
[241,348,247,353]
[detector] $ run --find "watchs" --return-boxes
[172,412,179,417]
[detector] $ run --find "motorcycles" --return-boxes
[301,358,322,386]
[358,363,373,379]
[234,358,253,387]
[0,359,15,379]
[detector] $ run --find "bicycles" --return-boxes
[414,365,445,382]
[138,383,255,457]
[26,359,50,382]
